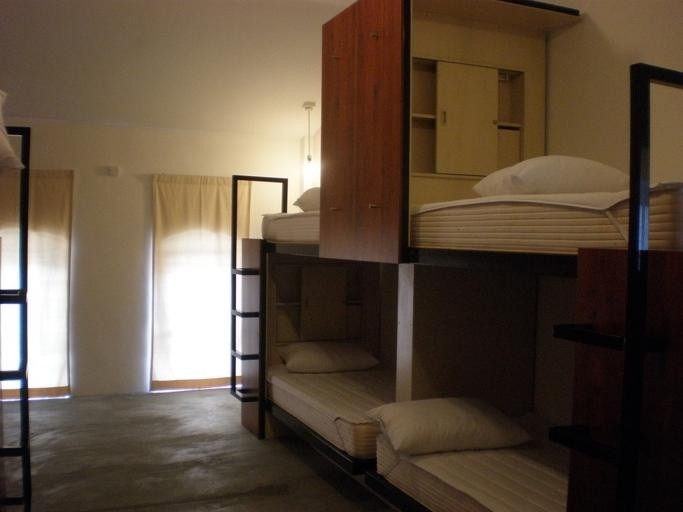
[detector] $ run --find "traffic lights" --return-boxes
[274,342,381,373]
[471,153,631,197]
[368,395,533,455]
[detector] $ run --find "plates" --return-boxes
[271,262,366,344]
[408,54,523,176]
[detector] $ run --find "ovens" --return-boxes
[256,186,682,511]
